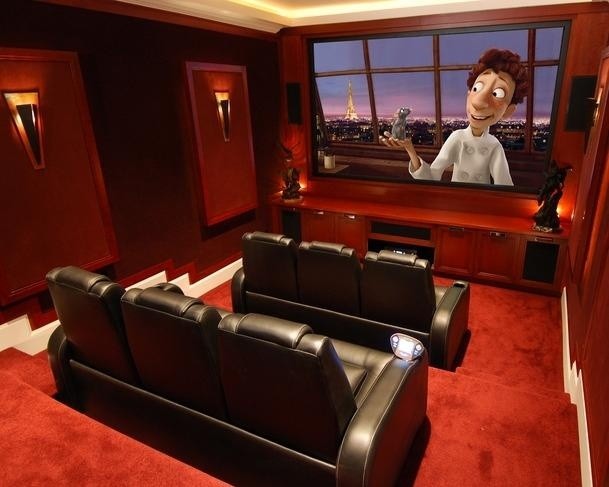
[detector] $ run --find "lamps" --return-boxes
[215,89,234,143]
[1,86,48,171]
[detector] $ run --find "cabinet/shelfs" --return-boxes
[300,207,369,260]
[436,224,520,285]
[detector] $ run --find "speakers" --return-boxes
[286,83,304,125]
[563,75,599,133]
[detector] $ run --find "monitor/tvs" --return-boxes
[307,18,571,198]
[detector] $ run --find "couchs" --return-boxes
[229,228,470,373]
[37,263,430,484]
